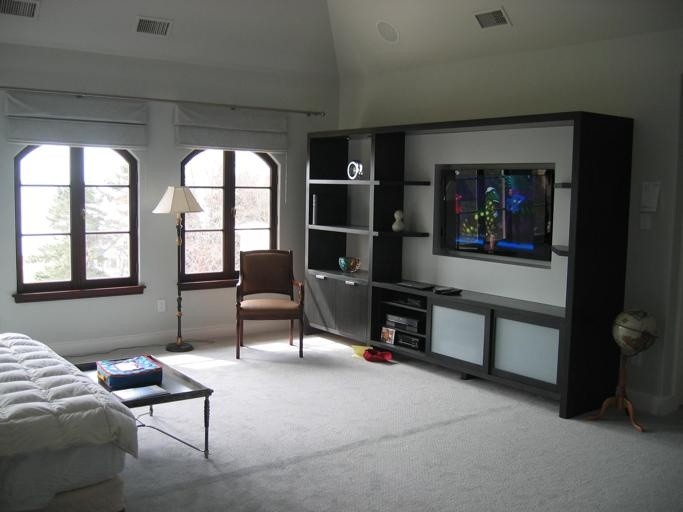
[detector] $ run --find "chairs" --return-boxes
[232,246,306,362]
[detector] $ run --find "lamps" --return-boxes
[149,185,207,355]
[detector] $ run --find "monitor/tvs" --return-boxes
[440,168,554,261]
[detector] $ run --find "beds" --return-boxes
[0,331,142,510]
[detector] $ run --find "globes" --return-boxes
[585,305,665,433]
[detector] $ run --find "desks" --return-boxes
[74,352,216,462]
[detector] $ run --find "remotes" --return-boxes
[441,289,462,295]
[433,288,451,294]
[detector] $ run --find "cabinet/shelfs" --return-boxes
[305,270,369,342]
[369,111,635,420]
[306,125,403,281]
